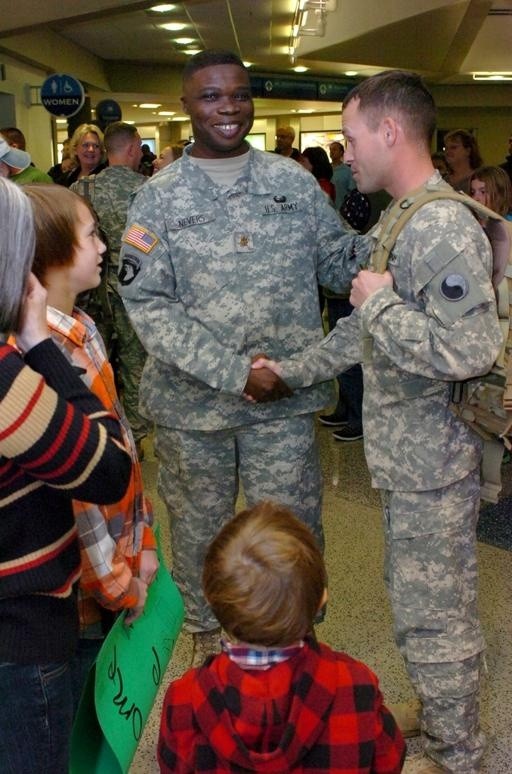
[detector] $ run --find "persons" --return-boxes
[2,175,159,773]
[117,47,371,618]
[1,48,511,441]
[159,499,406,774]
[242,71,504,771]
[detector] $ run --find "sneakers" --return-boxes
[383,697,423,740]
[318,413,350,425]
[399,754,450,774]
[332,426,363,441]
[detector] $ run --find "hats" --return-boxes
[0,136,33,170]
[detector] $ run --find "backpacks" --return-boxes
[339,187,371,230]
[446,219,512,442]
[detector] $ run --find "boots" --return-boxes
[190,628,224,669]
